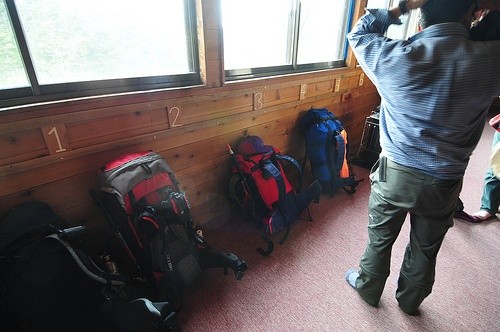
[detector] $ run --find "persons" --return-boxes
[459,1,500,223]
[345,0,500,314]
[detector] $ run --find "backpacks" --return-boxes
[227,133,322,255]
[0,202,166,332]
[89,148,250,320]
[302,107,363,195]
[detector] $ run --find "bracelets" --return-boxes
[398,0,410,15]
